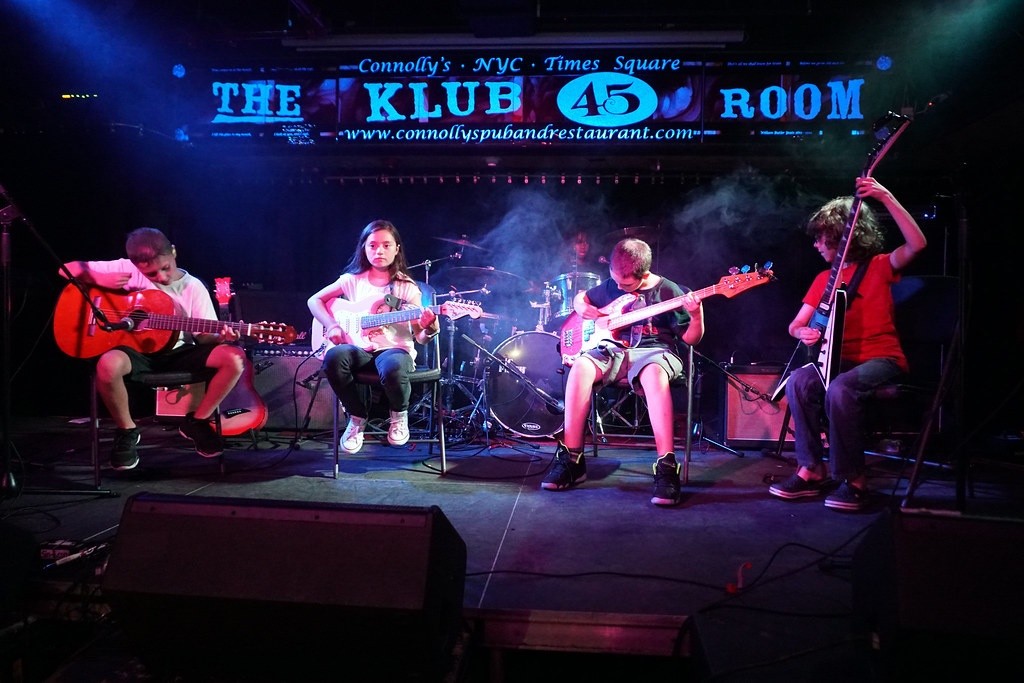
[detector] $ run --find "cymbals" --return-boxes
[602,225,661,244]
[443,266,531,289]
[435,235,490,255]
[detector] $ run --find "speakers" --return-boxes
[851,509,1024,653]
[104,491,467,683]
[155,381,206,425]
[250,344,347,432]
[470,607,718,683]
[718,362,803,451]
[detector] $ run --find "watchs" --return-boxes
[424,327,440,339]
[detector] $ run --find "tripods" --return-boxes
[405,316,483,435]
[678,340,770,458]
[444,334,558,451]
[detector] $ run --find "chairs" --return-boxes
[333,270,447,479]
[592,283,702,484]
[90,258,227,491]
[818,276,974,510]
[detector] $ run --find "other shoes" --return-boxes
[769,473,821,498]
[824,482,869,510]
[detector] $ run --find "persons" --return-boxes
[541,238,704,504]
[58,228,246,472]
[561,231,591,272]
[307,221,440,456]
[769,176,927,510]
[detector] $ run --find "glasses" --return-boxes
[576,239,589,245]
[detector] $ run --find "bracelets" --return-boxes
[327,323,339,333]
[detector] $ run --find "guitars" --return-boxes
[561,262,777,368]
[209,277,267,436]
[312,296,482,363]
[771,111,909,400]
[54,278,298,358]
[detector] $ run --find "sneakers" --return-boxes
[179,411,225,457]
[540,440,587,489]
[651,452,682,504]
[387,409,410,445]
[109,427,141,471]
[340,412,368,455]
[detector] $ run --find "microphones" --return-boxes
[554,399,565,414]
[101,318,135,331]
[295,380,316,391]
[163,384,185,391]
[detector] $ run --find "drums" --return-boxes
[483,331,592,438]
[550,272,602,326]
[445,375,480,412]
